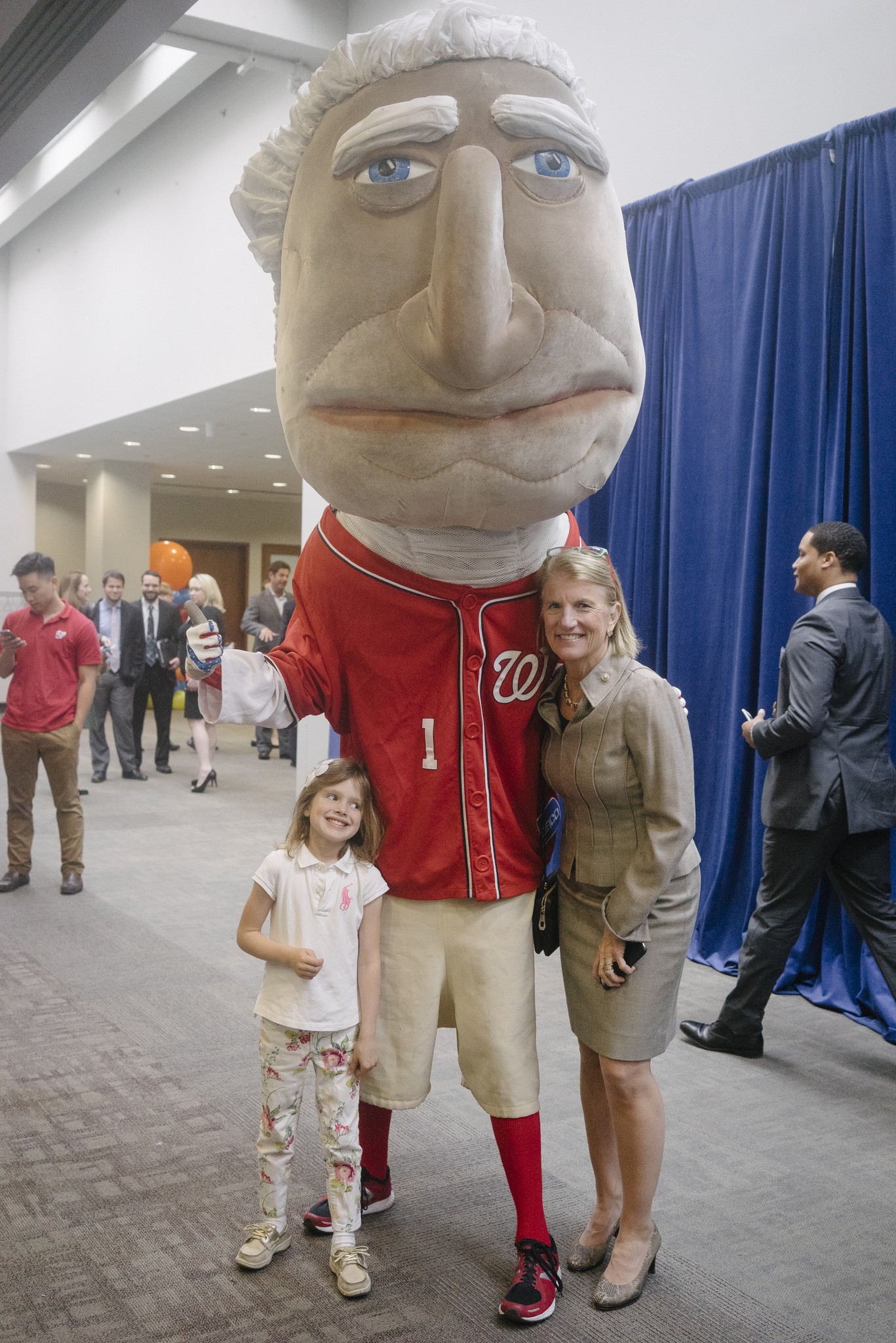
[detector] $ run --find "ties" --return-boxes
[109,606,120,673]
[145,606,157,668]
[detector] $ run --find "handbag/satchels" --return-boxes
[533,871,560,956]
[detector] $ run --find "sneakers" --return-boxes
[302,1164,395,1234]
[329,1243,372,1297]
[499,1233,563,1323]
[234,1222,292,1269]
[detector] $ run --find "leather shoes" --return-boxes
[91,771,105,781]
[0,872,28,893]
[62,871,82,893]
[679,1020,764,1059]
[122,769,149,780]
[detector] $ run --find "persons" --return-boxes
[0,553,101,894]
[241,561,296,760]
[681,523,896,1061]
[175,573,218,791]
[531,546,703,1312]
[229,0,688,1327]
[251,580,280,748]
[141,582,182,751]
[90,569,148,781]
[129,569,184,775]
[56,573,113,795]
[235,754,377,1296]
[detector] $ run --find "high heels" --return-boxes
[192,779,213,785]
[567,1217,620,1272]
[592,1221,663,1308]
[191,769,218,792]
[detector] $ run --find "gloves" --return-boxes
[183,600,223,681]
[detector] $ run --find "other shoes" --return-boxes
[259,752,269,759]
[157,764,172,773]
[169,742,180,752]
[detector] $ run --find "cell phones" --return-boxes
[0,629,20,643]
[742,709,753,721]
[598,939,646,990]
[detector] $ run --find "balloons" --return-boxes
[149,540,193,592]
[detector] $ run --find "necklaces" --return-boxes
[564,675,580,712]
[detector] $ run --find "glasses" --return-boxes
[545,546,617,587]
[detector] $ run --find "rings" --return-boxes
[600,964,613,970]
